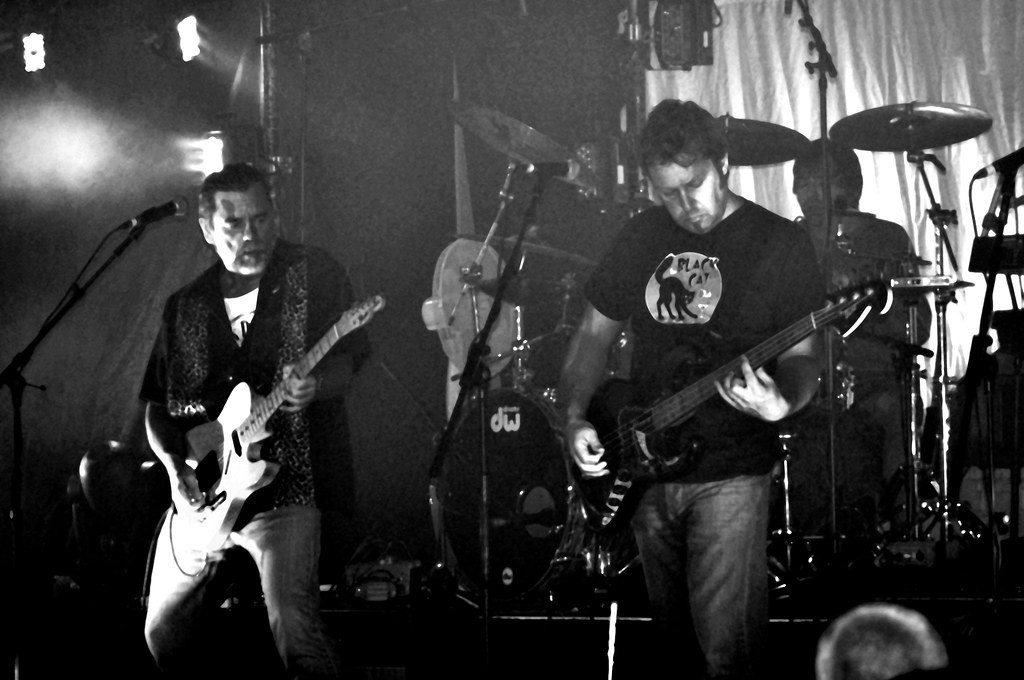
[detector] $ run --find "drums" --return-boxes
[428,386,594,601]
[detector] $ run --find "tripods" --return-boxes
[764,129,1007,604]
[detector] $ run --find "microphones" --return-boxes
[974,146,1024,179]
[522,158,578,181]
[120,196,189,231]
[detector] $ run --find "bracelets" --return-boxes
[315,369,329,398]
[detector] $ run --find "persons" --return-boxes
[557,98,953,680]
[140,164,381,680]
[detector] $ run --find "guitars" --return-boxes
[188,288,388,550]
[569,273,897,529]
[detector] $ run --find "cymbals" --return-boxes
[460,233,598,268]
[716,119,809,164]
[828,101,993,152]
[448,100,601,188]
[816,239,929,266]
